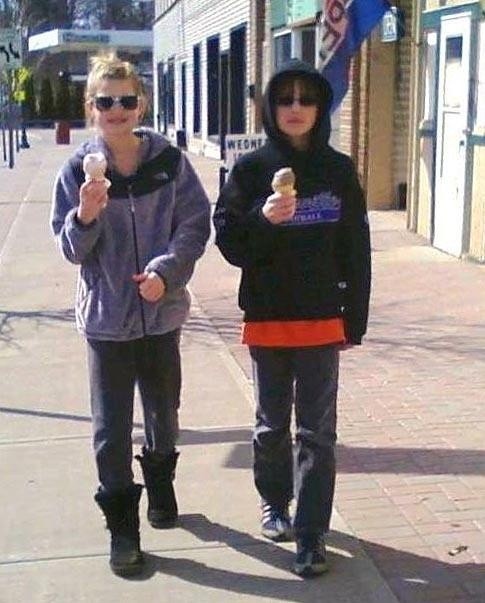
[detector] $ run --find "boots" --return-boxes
[136,449,181,528]
[94,483,147,576]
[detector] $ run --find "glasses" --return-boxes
[87,95,139,114]
[274,93,317,108]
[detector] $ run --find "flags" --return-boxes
[315,0,393,115]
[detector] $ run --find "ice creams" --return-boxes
[272,167,296,216]
[83,153,107,208]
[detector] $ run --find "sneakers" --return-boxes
[292,523,327,576]
[258,500,293,541]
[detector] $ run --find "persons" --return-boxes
[214,56,374,577]
[47,51,212,579]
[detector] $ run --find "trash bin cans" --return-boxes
[54,120,70,144]
